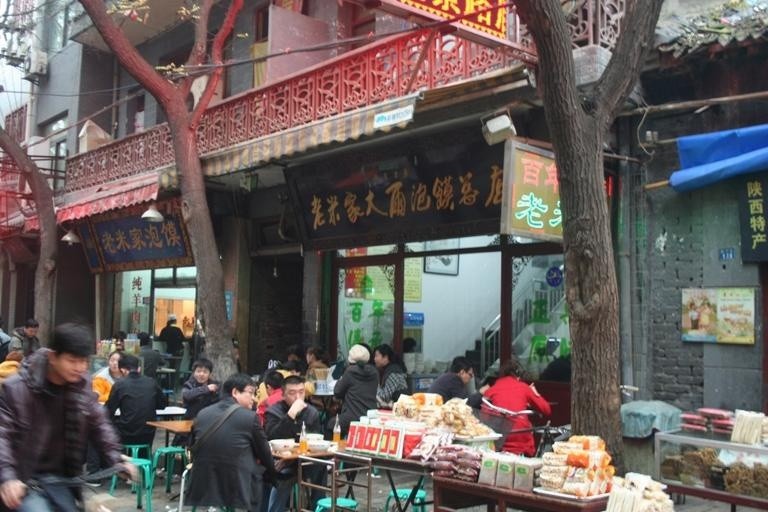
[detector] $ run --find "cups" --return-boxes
[315,380,328,393]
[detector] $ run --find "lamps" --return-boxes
[239,171,260,194]
[141,200,164,223]
[60,221,81,247]
[478,107,518,145]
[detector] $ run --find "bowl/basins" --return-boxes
[271,439,295,453]
[303,433,324,440]
[307,441,330,452]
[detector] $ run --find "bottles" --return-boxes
[332,415,341,442]
[300,425,307,454]
[96,337,141,356]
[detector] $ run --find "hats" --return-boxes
[347,344,372,366]
[166,314,177,321]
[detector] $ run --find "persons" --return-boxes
[690,299,712,330]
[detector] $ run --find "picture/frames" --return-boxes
[422,238,460,276]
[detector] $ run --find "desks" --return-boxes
[146,368,196,447]
[312,380,336,422]
[265,440,337,459]
[666,482,767,511]
[431,470,617,511]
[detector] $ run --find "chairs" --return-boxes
[292,477,358,511]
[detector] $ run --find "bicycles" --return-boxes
[454,382,570,511]
[11,462,141,512]
[618,385,639,398]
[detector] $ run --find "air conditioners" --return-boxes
[23,48,48,77]
[184,74,223,112]
[14,135,53,199]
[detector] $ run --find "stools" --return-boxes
[382,488,428,512]
[107,442,193,512]
